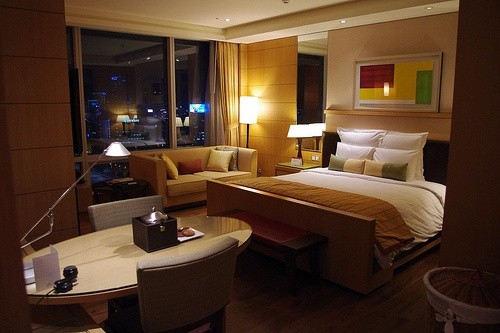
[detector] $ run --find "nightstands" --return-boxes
[273,160,322,177]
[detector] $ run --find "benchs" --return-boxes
[218,209,329,294]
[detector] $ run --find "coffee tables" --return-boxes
[91,178,151,204]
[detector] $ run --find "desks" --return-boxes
[22,214,253,333]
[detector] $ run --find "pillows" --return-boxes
[327,125,429,182]
[151,148,239,180]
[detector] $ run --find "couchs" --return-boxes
[128,144,258,212]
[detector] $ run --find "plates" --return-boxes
[177,226,205,243]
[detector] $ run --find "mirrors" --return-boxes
[295,30,330,152]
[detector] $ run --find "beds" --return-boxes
[206,109,453,296]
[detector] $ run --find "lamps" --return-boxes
[115,114,132,137]
[176,116,189,145]
[287,124,313,165]
[309,123,326,151]
[20,141,132,248]
[239,95,257,147]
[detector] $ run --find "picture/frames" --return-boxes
[353,51,443,113]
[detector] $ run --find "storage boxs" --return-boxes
[133,210,179,255]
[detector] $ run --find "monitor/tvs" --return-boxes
[189,103,205,113]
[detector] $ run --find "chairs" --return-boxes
[87,195,239,333]
